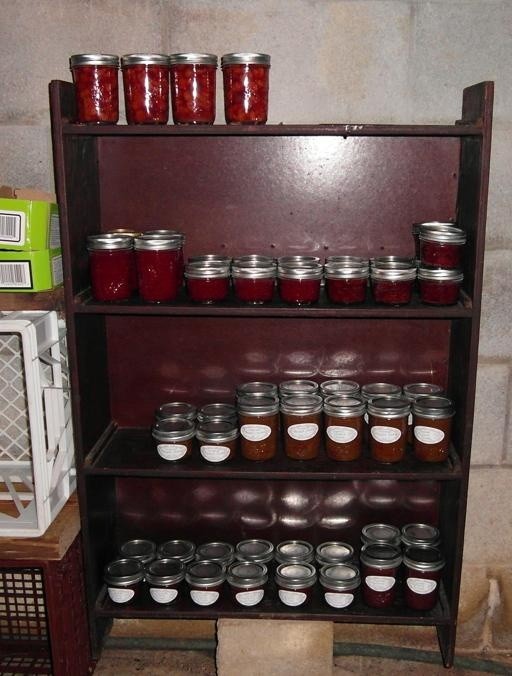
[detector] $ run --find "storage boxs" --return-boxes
[0,185,64,294]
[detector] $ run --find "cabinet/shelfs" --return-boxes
[48,78,494,669]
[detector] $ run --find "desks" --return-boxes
[0,480,96,675]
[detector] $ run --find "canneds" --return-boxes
[417,267,464,305]
[361,382,413,462]
[359,526,403,610]
[278,381,324,461]
[324,255,370,305]
[412,222,466,270]
[153,401,198,462]
[170,53,217,124]
[403,383,452,462]
[277,255,324,305]
[226,539,274,610]
[120,55,170,125]
[221,53,270,123]
[103,539,157,611]
[71,54,120,124]
[87,228,185,305]
[194,403,240,462]
[185,542,235,610]
[183,254,232,305]
[400,525,444,611]
[145,540,196,610]
[273,540,317,610]
[230,254,278,305]
[370,255,417,305]
[235,382,280,462]
[315,541,362,610]
[320,380,367,462]
